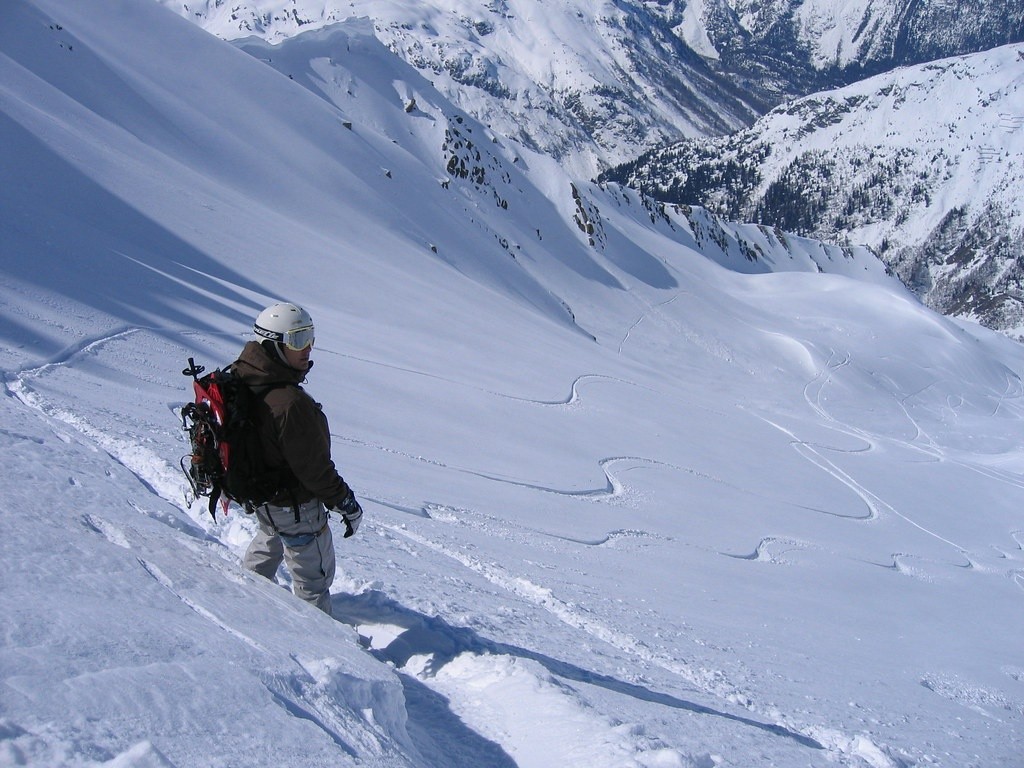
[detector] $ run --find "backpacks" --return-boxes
[193,371,299,524]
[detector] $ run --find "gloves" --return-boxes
[324,483,363,538]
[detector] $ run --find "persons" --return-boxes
[229,302,363,616]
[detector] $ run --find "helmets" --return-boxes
[255,303,313,345]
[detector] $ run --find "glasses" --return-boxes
[253,323,315,351]
